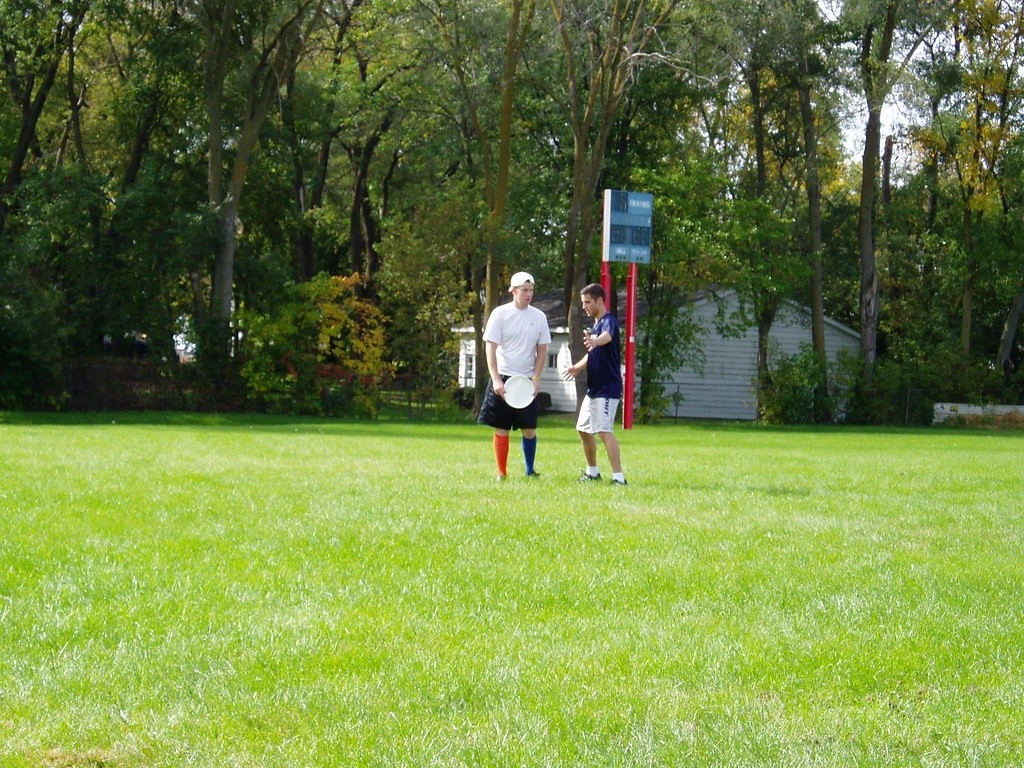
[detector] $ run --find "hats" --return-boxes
[508,272,536,292]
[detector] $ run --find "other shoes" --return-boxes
[529,472,539,478]
[610,478,627,486]
[577,473,603,483]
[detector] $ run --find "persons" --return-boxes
[562,283,626,486]
[478,272,552,482]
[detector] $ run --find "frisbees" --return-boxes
[503,374,535,410]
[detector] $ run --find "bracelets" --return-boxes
[533,377,540,381]
[580,363,586,368]
